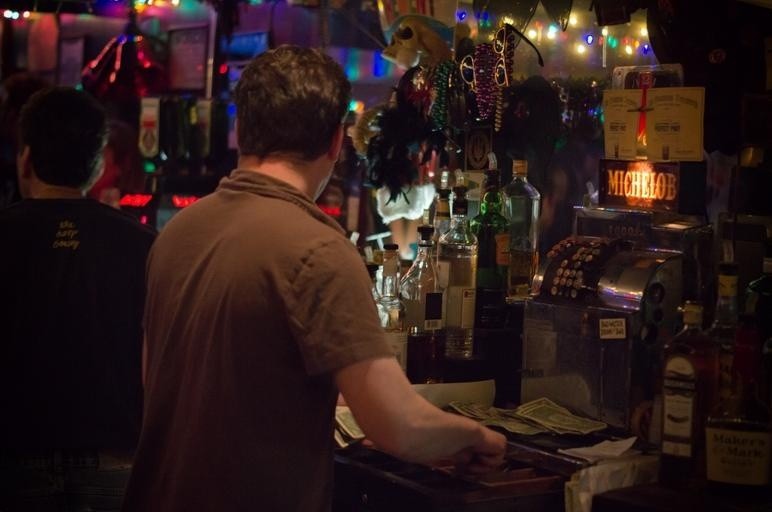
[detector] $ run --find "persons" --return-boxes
[2,88,160,510]
[122,42,510,511]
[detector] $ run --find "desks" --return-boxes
[324,371,696,512]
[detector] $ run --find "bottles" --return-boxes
[355,155,541,382]
[656,242,772,512]
